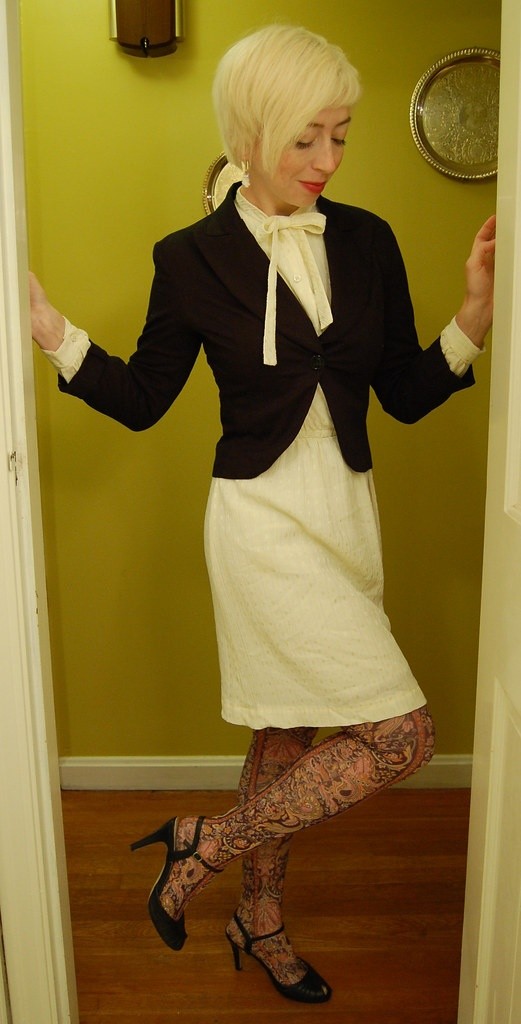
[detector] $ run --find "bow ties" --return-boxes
[237,190,334,367]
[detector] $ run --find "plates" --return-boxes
[410,47,499,179]
[201,151,244,215]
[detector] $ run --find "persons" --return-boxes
[30,26,497,1002]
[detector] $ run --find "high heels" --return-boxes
[130,816,224,951]
[225,908,332,1004]
